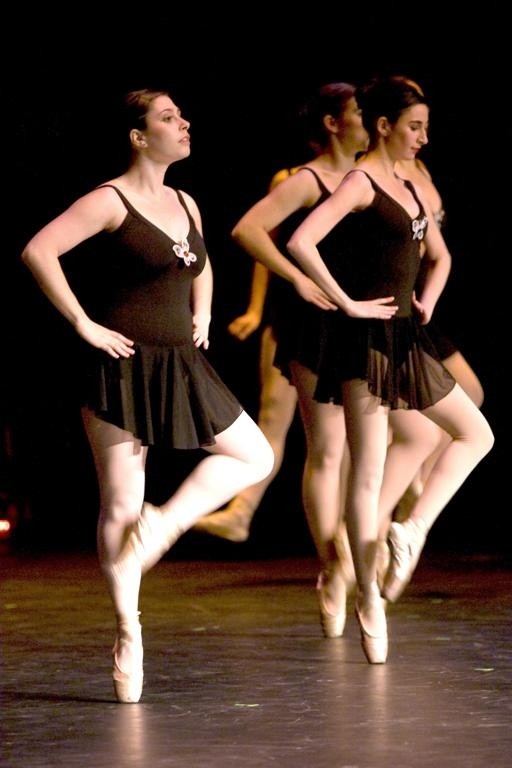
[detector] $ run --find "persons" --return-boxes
[23,89,271,703]
[193,76,495,665]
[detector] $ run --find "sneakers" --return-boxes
[107,502,172,578]
[112,629,145,703]
[197,495,255,544]
[316,569,347,639]
[384,521,415,603]
[353,593,388,666]
